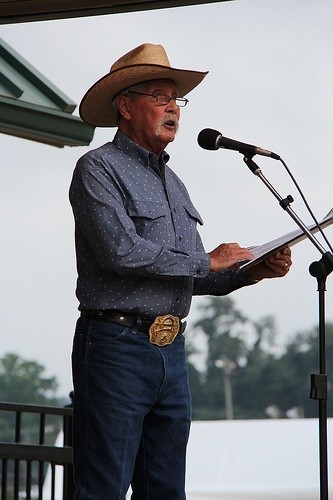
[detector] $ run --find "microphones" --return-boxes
[198,128,280,160]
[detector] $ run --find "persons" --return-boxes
[67,43,292,500]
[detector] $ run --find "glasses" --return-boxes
[125,91,189,107]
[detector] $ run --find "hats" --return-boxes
[79,43,209,127]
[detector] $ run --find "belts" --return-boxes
[81,309,186,346]
